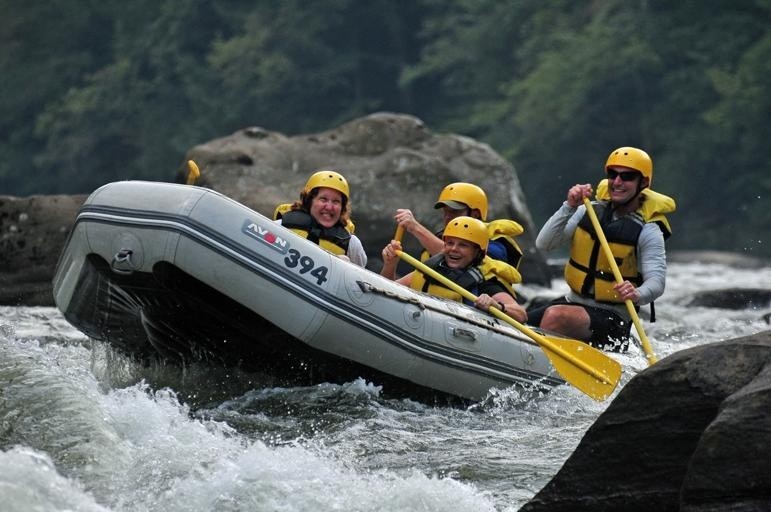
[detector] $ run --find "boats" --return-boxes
[52,181,652,411]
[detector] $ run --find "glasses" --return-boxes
[607,168,643,182]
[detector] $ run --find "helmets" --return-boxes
[303,170,351,204]
[605,146,654,189]
[433,182,489,223]
[442,215,489,254]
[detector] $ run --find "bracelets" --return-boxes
[496,300,507,314]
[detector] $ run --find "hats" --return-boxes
[434,201,468,211]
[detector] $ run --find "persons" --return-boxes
[392,177,525,276]
[528,145,677,354]
[267,168,368,267]
[380,215,530,335]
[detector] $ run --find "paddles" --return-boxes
[394,251,621,402]
